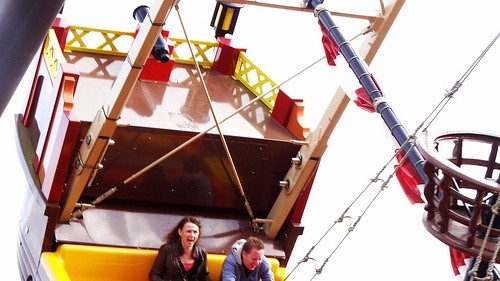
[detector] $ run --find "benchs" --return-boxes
[31,243,288,281]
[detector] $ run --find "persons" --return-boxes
[219,235,274,281]
[148,216,212,281]
